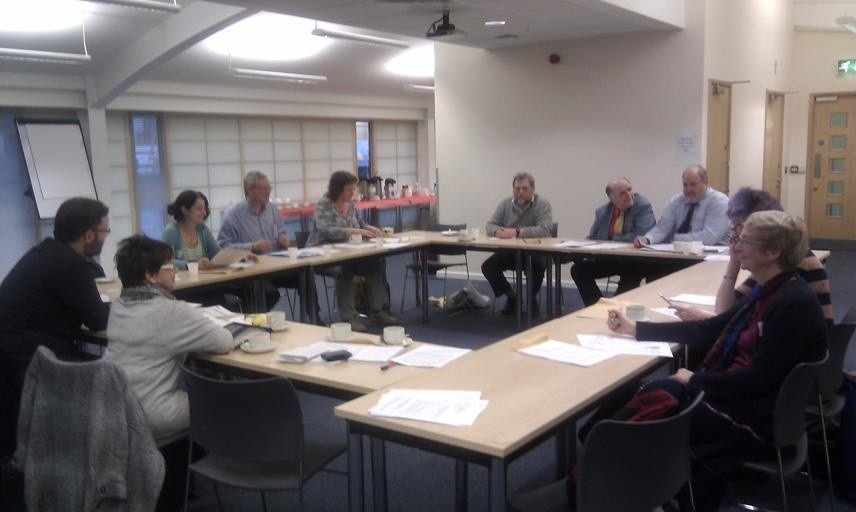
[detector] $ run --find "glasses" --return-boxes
[91,227,110,233]
[258,186,272,191]
[737,235,769,246]
[160,263,176,272]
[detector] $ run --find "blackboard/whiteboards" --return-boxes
[15,117,98,224]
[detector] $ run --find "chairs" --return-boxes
[819,303,855,496]
[14,343,224,510]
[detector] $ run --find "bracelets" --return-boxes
[724,277,739,280]
[515,227,520,238]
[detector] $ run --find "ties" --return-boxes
[613,209,625,235]
[677,203,697,233]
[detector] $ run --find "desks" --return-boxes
[279,192,438,233]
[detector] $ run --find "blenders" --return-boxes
[367,176,383,200]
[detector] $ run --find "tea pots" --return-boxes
[384,178,399,200]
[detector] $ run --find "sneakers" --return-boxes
[369,310,398,324]
[501,299,517,315]
[343,321,366,331]
[526,295,539,317]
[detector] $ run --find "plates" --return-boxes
[95,277,115,283]
[240,323,412,354]
[441,230,457,236]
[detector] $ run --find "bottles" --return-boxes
[401,185,408,198]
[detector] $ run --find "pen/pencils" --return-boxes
[381,362,396,370]
[657,292,673,306]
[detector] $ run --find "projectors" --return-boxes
[421,26,467,43]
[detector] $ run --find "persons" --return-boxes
[1,198,111,484]
[668,187,835,407]
[482,172,551,316]
[218,171,326,326]
[570,178,655,310]
[303,171,398,331]
[105,232,232,497]
[581,212,830,512]
[636,164,728,282]
[160,189,245,312]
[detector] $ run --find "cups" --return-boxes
[458,230,471,241]
[187,262,198,277]
[471,229,480,240]
[349,234,362,243]
[690,241,703,255]
[375,231,383,247]
[673,241,689,251]
[242,311,406,349]
[625,305,645,320]
[288,247,298,259]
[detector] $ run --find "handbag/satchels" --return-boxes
[463,282,490,308]
[567,390,681,510]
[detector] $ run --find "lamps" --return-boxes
[36,0,182,19]
[402,85,437,97]
[0,17,92,67]
[311,18,410,49]
[834,10,855,37]
[225,52,328,88]
